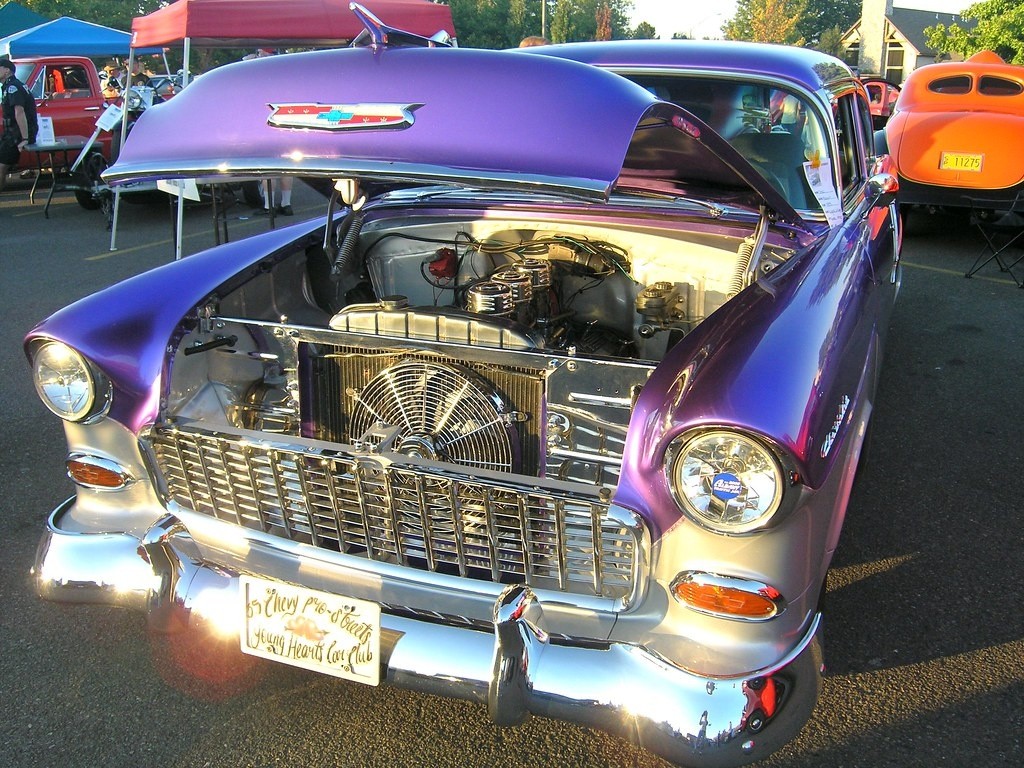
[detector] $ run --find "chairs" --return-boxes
[728,133,821,210]
[961,190,1024,289]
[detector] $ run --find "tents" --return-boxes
[8,16,175,90]
[111,0,457,261]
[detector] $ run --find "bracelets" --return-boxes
[22,138,28,141]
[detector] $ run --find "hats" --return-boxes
[0,59,16,73]
[104,60,121,71]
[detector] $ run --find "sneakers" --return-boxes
[276,203,294,216]
[253,207,277,218]
[20,169,37,179]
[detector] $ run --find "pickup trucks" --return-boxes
[0,54,179,175]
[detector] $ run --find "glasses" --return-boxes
[126,63,129,66]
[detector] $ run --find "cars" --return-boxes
[871,49,1024,237]
[19,30,906,768]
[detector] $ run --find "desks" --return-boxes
[22,135,106,220]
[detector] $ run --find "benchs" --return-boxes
[52,92,92,98]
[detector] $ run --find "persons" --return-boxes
[519,36,552,48]
[99,58,154,97]
[263,177,295,218]
[0,58,38,193]
